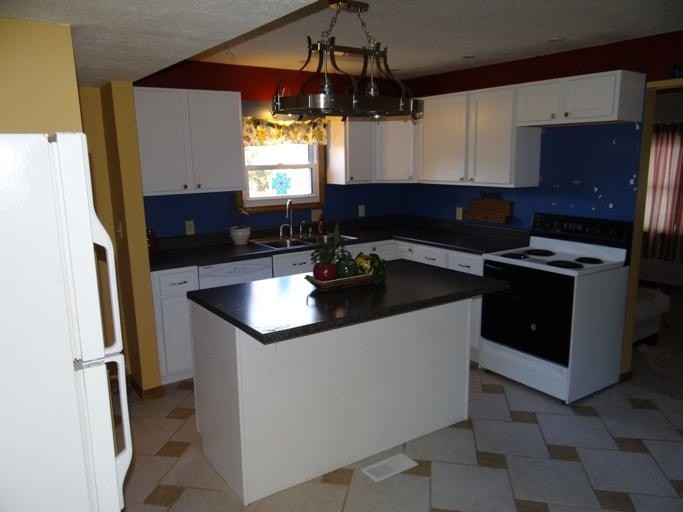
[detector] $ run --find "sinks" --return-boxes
[298,233,358,245]
[256,238,313,250]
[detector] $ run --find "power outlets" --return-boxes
[185,220,195,235]
[310,209,322,222]
[358,205,365,217]
[456,207,461,219]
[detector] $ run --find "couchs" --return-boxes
[633,286,669,347]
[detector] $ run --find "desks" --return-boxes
[186,258,511,507]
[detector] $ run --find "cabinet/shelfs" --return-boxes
[516,70,644,127]
[414,243,448,269]
[148,266,199,386]
[131,86,246,195]
[325,115,415,186]
[415,81,541,188]
[273,239,397,279]
[397,237,413,261]
[448,248,480,370]
[192,256,273,289]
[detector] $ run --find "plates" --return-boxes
[303,274,376,292]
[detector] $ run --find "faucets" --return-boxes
[286,199,292,238]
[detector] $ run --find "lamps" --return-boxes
[272,1,424,121]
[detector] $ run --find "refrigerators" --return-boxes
[0,130,134,512]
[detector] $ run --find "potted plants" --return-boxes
[224,198,260,245]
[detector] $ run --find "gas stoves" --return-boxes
[483,212,636,279]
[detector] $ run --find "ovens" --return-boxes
[473,259,631,405]
